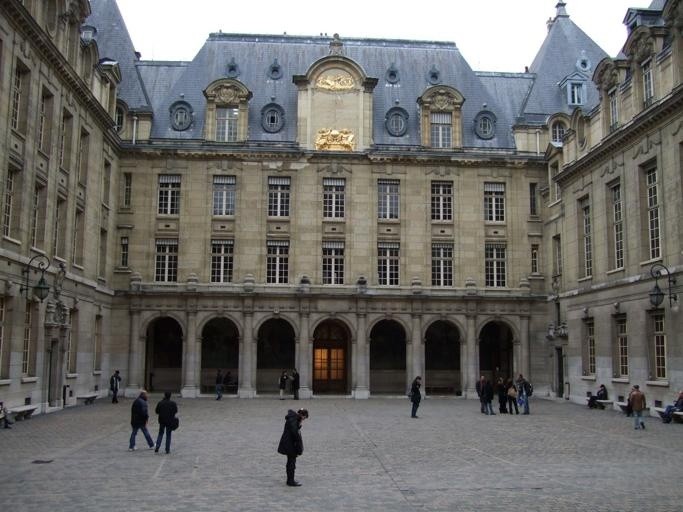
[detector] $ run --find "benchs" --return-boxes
[76,393,98,406]
[585,397,683,423]
[6,404,38,424]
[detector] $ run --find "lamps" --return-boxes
[20,255,50,304]
[648,262,677,311]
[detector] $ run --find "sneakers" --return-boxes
[286,480,303,487]
[634,421,645,430]
[481,408,529,415]
[128,443,171,454]
[411,415,419,418]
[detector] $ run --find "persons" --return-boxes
[84,385,99,406]
[0,401,14,429]
[658,391,683,424]
[110,369,121,404]
[277,408,309,486]
[289,368,300,400]
[127,388,156,451]
[279,371,288,400]
[631,384,645,430]
[410,375,422,419]
[316,128,355,146]
[223,371,232,392]
[154,390,178,455]
[475,373,534,415]
[215,368,222,400]
[626,388,633,416]
[585,384,608,409]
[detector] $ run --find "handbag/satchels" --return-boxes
[172,416,179,431]
[407,392,413,397]
